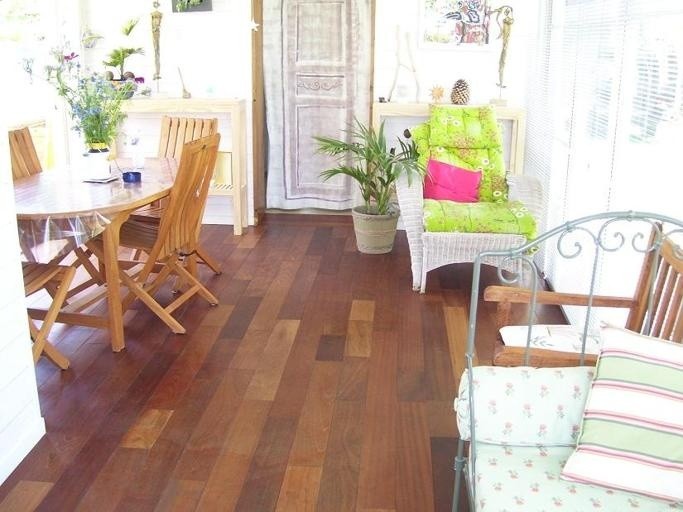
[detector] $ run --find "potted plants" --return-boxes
[306,111,438,256]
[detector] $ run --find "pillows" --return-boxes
[452,361,601,449]
[554,316,683,506]
[420,156,484,203]
[428,100,504,151]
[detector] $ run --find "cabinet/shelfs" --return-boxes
[67,97,250,238]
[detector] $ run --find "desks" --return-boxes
[12,153,199,355]
[365,100,530,201]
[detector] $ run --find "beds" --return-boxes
[451,207,683,510]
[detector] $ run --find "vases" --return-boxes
[81,140,109,150]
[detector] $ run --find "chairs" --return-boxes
[482,220,683,373]
[7,126,109,314]
[124,113,223,295]
[384,131,549,295]
[75,132,223,337]
[17,254,78,372]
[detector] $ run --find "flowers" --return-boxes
[22,16,147,161]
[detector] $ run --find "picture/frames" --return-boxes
[418,0,495,52]
